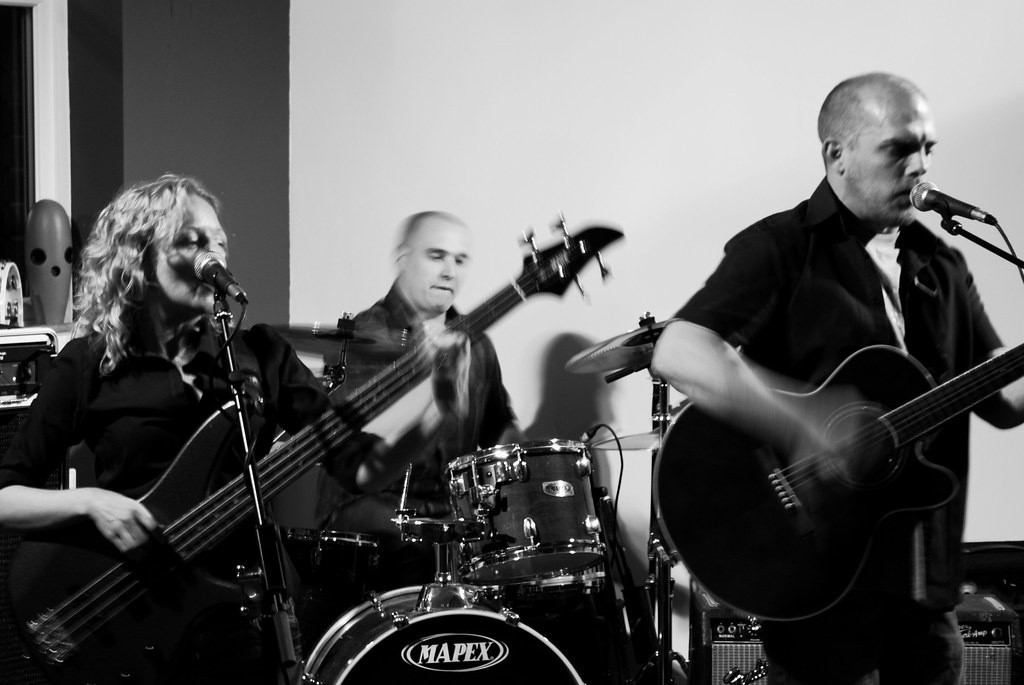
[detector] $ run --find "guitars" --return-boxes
[651,336,1024,626]
[9,214,624,685]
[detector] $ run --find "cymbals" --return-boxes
[266,324,411,360]
[565,317,674,375]
[591,427,664,451]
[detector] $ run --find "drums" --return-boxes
[271,517,437,653]
[441,436,611,586]
[298,578,587,685]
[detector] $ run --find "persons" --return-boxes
[649,74,1024,685]
[0,173,475,684]
[315,209,530,590]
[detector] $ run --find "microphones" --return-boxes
[580,426,599,443]
[909,181,998,226]
[195,252,248,307]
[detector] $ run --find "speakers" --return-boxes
[687,577,1024,685]
